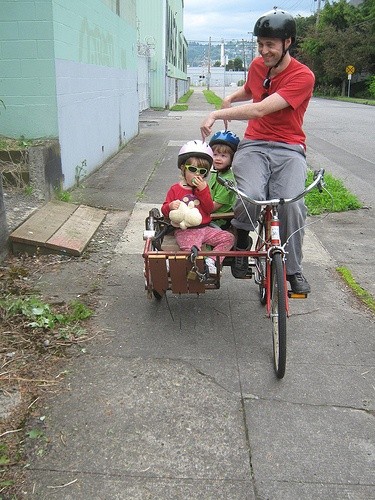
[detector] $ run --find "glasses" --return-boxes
[260,78,270,101]
[183,163,209,175]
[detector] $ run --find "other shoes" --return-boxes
[231,234,252,278]
[204,258,222,275]
[286,272,311,293]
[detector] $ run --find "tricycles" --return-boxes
[142,168,335,379]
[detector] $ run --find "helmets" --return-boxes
[209,130,240,153]
[253,9,297,39]
[178,140,214,168]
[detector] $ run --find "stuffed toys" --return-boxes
[168,199,203,231]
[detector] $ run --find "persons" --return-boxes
[161,139,237,276]
[198,8,313,295]
[206,130,239,231]
